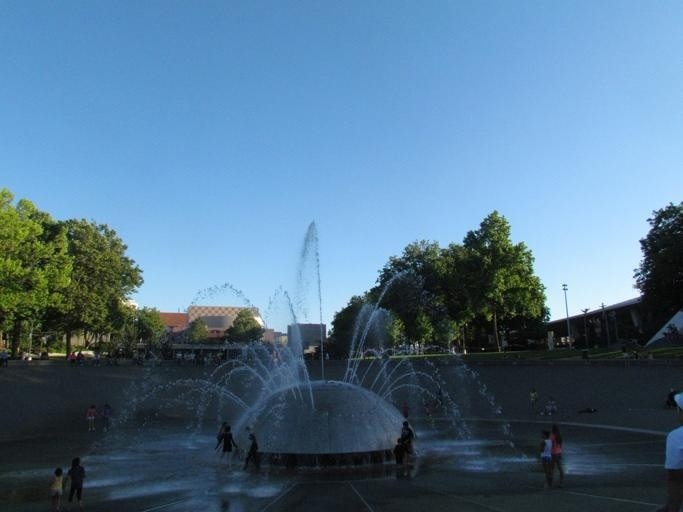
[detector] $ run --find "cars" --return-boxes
[20,351,48,360]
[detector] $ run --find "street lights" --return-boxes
[581,308,590,349]
[600,302,610,352]
[562,284,572,353]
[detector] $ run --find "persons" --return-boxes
[527,388,539,413]
[217,421,228,449]
[242,425,258,469]
[577,406,599,415]
[213,424,238,472]
[550,422,565,488]
[1,337,286,372]
[539,427,553,485]
[539,396,558,417]
[84,404,99,431]
[662,386,678,409]
[48,467,63,512]
[63,456,87,511]
[655,391,682,511]
[387,385,444,481]
[101,399,111,430]
